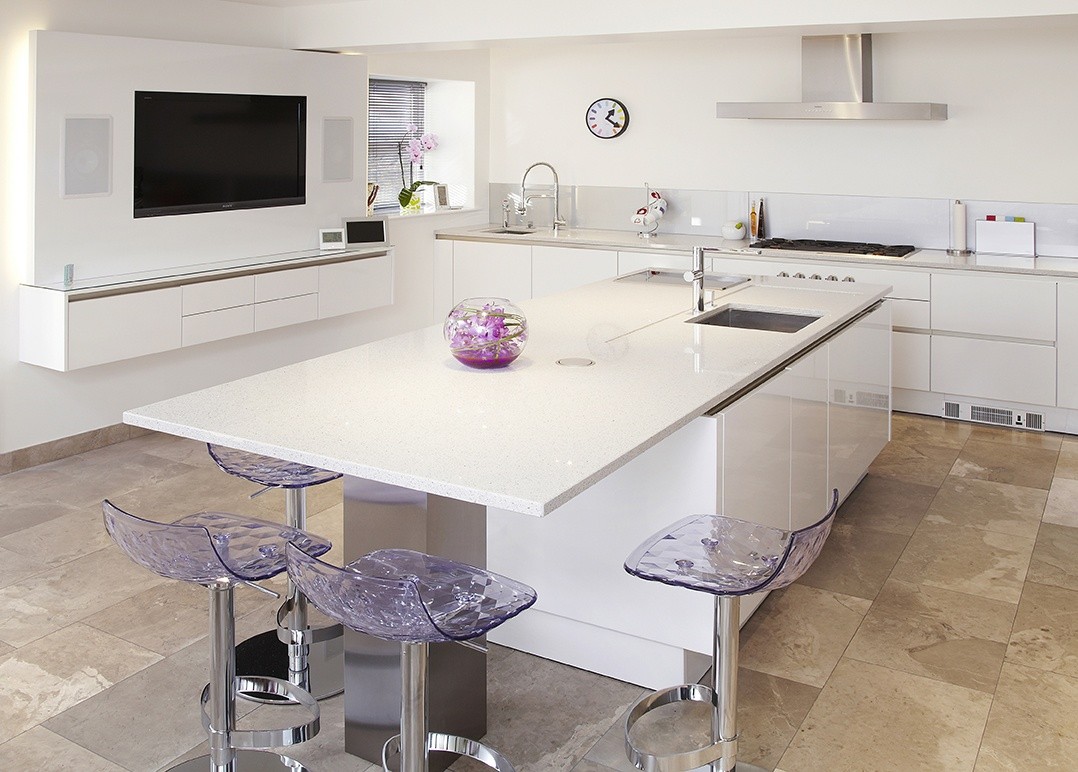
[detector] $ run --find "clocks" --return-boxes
[585,98,629,139]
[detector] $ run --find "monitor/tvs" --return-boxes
[135,91,306,218]
[342,215,390,248]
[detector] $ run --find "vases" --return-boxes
[399,191,421,215]
[442,295,529,370]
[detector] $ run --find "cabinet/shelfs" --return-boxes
[19,243,397,374]
[930,272,1057,342]
[453,239,692,309]
[931,335,1057,408]
[1056,281,1078,411]
[489,296,894,694]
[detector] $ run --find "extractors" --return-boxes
[717,33,948,120]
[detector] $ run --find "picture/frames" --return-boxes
[58,112,112,198]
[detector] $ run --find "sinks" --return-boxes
[480,228,540,238]
[686,300,840,335]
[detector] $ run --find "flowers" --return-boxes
[395,122,442,208]
[448,302,528,367]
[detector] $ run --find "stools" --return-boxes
[101,496,335,772]
[282,538,539,772]
[206,439,346,707]
[615,488,841,772]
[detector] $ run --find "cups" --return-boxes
[632,208,650,226]
[651,192,667,216]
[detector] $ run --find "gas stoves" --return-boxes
[721,235,922,281]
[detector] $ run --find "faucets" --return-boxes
[683,242,724,313]
[517,159,569,232]
